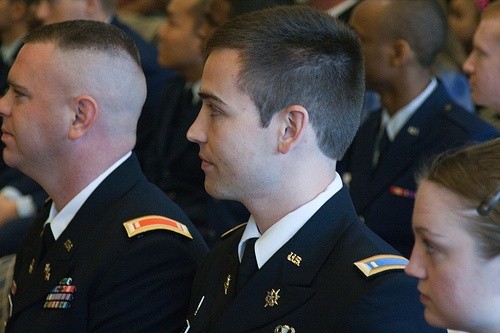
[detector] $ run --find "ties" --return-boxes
[171,88,194,130]
[235,238,260,295]
[376,125,390,159]
[32,223,56,274]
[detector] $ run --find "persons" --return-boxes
[177,6,449,333]
[333,0,500,264]
[403,137,498,333]
[1,1,33,96]
[1,0,156,256]
[156,2,251,251]
[99,0,500,131]
[1,20,212,333]
